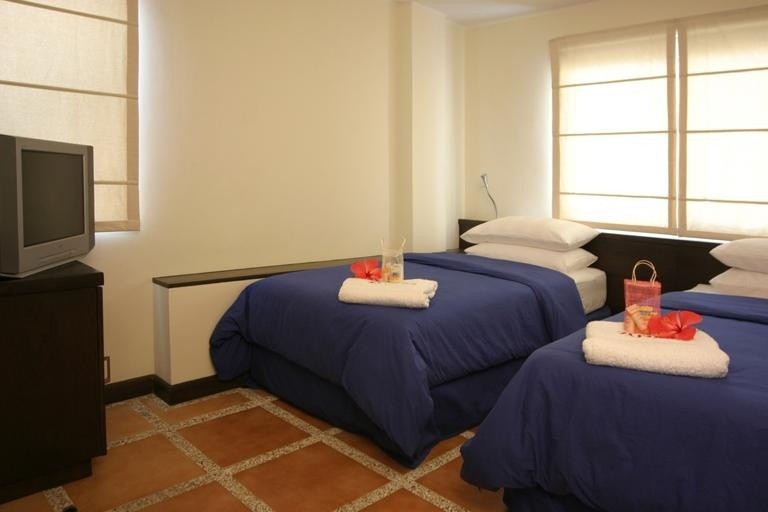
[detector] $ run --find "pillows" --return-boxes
[461,216,600,273]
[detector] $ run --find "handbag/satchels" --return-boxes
[625,280,661,312]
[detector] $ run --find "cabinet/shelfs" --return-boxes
[0,261,110,504]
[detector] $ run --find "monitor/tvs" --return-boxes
[0,131,97,279]
